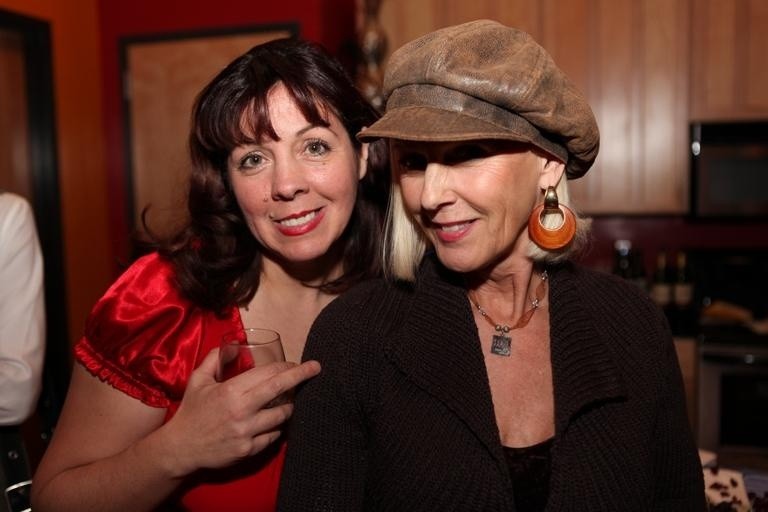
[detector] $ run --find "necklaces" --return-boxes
[466,269,548,357]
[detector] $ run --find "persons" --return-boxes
[274,19,707,512]
[29,39,391,511]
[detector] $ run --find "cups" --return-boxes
[215,328,287,411]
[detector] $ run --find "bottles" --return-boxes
[609,237,698,337]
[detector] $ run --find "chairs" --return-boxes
[674,338,722,448]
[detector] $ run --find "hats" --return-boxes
[356,18,599,180]
